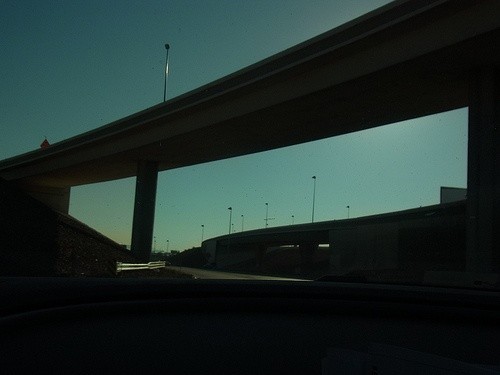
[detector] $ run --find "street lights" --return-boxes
[228,206,234,234]
[166,239,169,252]
[266,203,268,228]
[347,206,350,219]
[201,224,204,242]
[292,215,294,224]
[312,175,316,223]
[163,43,169,103]
[241,214,244,232]
